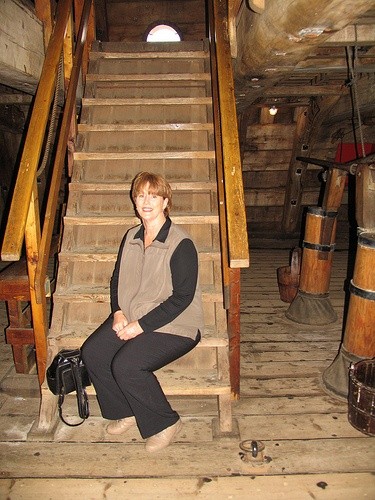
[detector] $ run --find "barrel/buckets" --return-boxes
[347,359,375,437]
[277,265,301,302]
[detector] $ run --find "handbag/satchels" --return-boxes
[46,349,93,427]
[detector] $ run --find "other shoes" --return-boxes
[144,419,184,454]
[106,416,137,435]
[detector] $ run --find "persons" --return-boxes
[81,172,202,455]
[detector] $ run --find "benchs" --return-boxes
[0,234,59,374]
